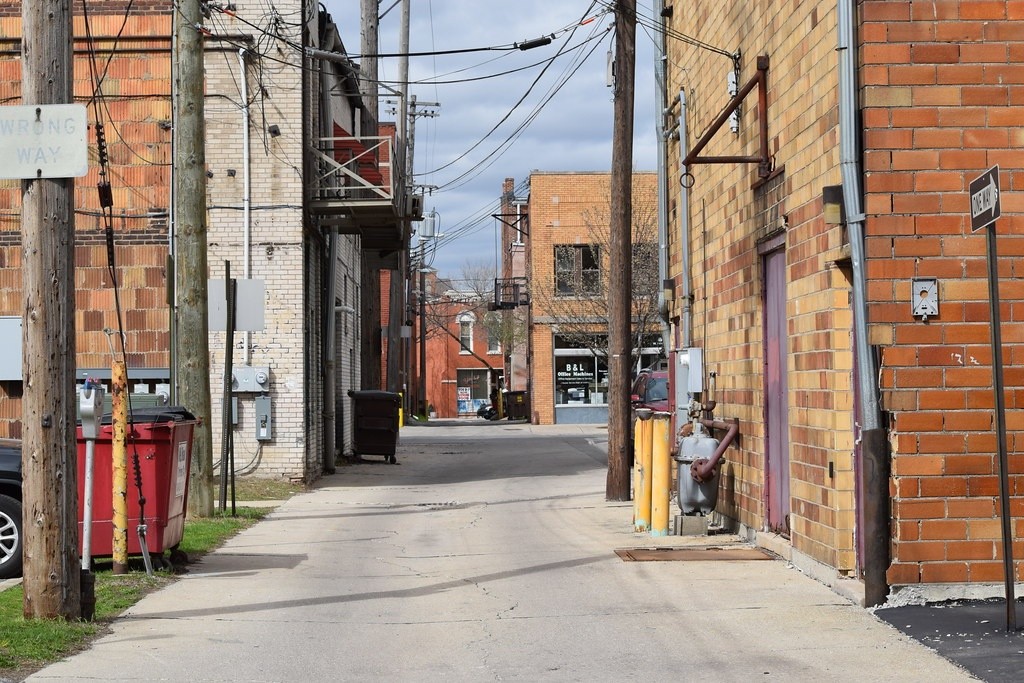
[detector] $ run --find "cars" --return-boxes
[0,446,22,581]
[477,404,495,420]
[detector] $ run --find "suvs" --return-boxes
[630,368,670,467]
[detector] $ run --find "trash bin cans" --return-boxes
[347,388,403,464]
[492,390,527,420]
[77,405,200,573]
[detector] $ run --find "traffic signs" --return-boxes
[970,166,1001,233]
